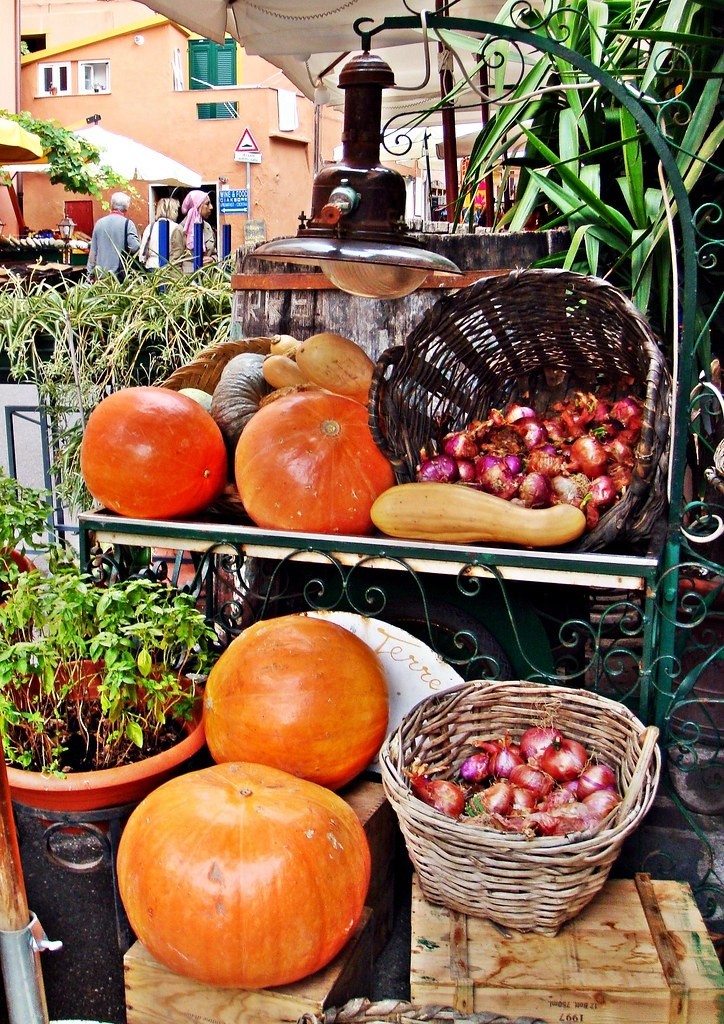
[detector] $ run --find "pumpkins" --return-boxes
[80,333,586,989]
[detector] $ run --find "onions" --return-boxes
[416,391,643,530]
[406,726,622,836]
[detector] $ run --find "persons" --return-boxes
[170,190,218,279]
[139,198,185,293]
[85,192,139,284]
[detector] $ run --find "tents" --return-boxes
[134,0,546,220]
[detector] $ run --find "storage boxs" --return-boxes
[121,864,724,1023]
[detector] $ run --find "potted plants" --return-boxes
[0,467,56,655]
[1,548,210,966]
